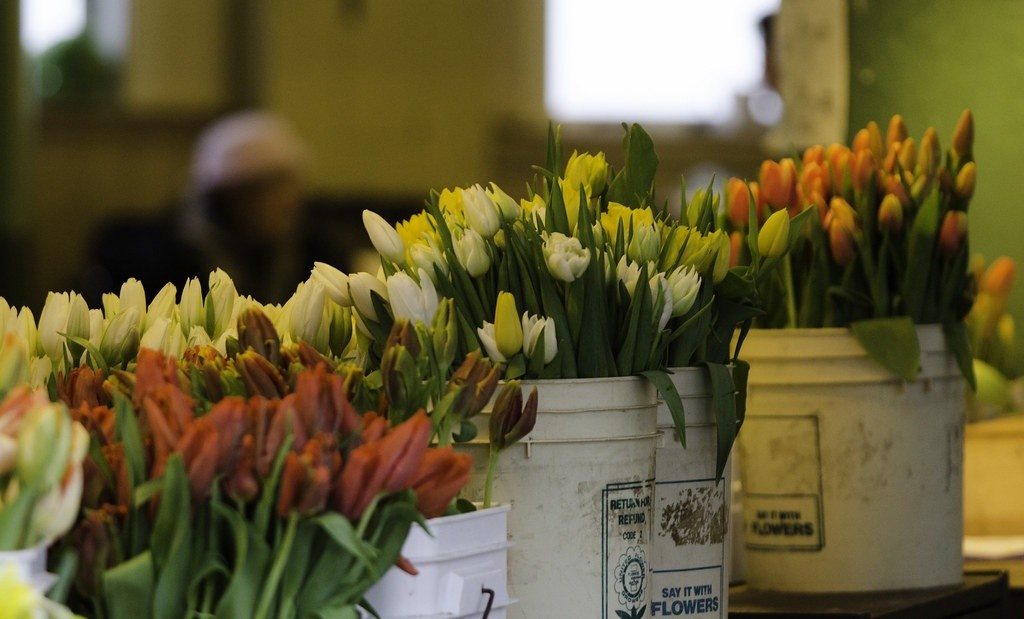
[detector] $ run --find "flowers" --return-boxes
[0,106,983,619]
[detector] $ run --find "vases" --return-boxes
[726,321,967,598]
[646,363,734,619]
[353,497,523,619]
[427,372,667,618]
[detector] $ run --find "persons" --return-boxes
[181,107,338,299]
